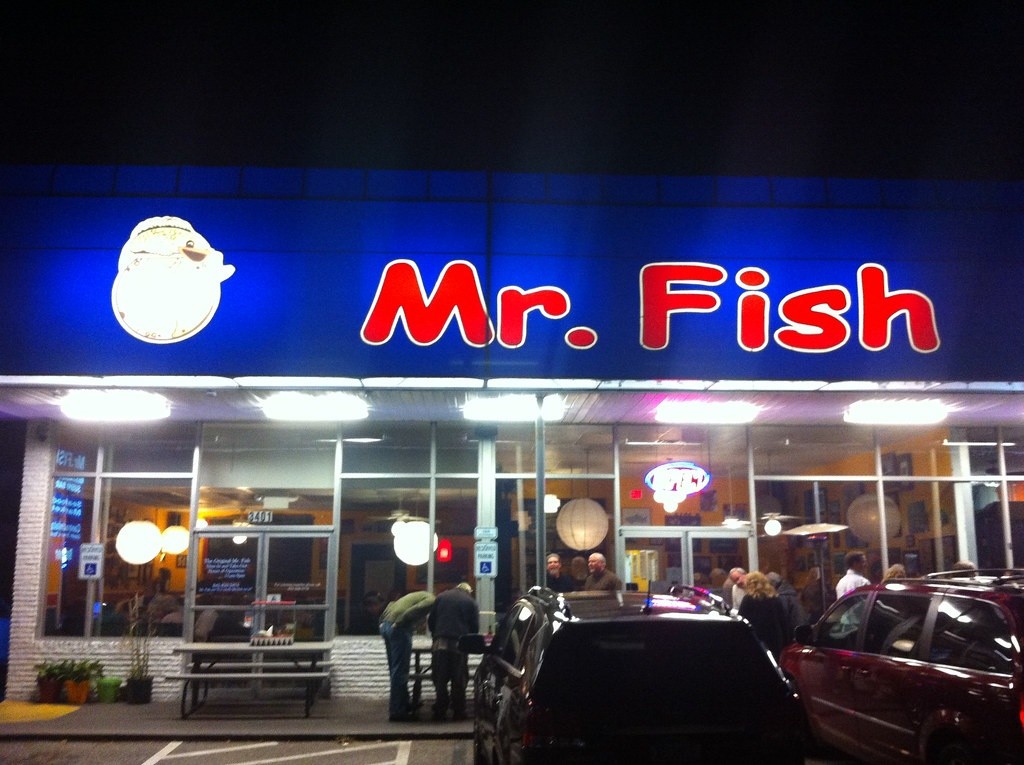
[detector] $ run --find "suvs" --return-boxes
[457,583,805,765]
[778,567,1022,765]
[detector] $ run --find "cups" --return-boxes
[267,594,281,602]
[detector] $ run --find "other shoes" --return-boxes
[431,703,448,714]
[452,711,469,719]
[405,702,423,712]
[389,712,419,722]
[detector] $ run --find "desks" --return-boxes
[173,642,333,671]
[411,641,433,670]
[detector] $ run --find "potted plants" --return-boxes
[62,659,104,704]
[35,659,70,703]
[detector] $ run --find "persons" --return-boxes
[729,567,809,661]
[835,552,872,600]
[1003,568,1024,576]
[585,553,622,591]
[378,591,435,722]
[881,564,907,579]
[710,568,735,607]
[546,553,574,592]
[808,567,820,580]
[948,561,977,577]
[150,569,170,594]
[428,582,480,720]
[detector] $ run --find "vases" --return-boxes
[127,675,154,704]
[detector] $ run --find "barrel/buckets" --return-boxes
[96,679,122,703]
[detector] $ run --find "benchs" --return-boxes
[188,662,334,702]
[407,661,481,717]
[165,673,330,718]
